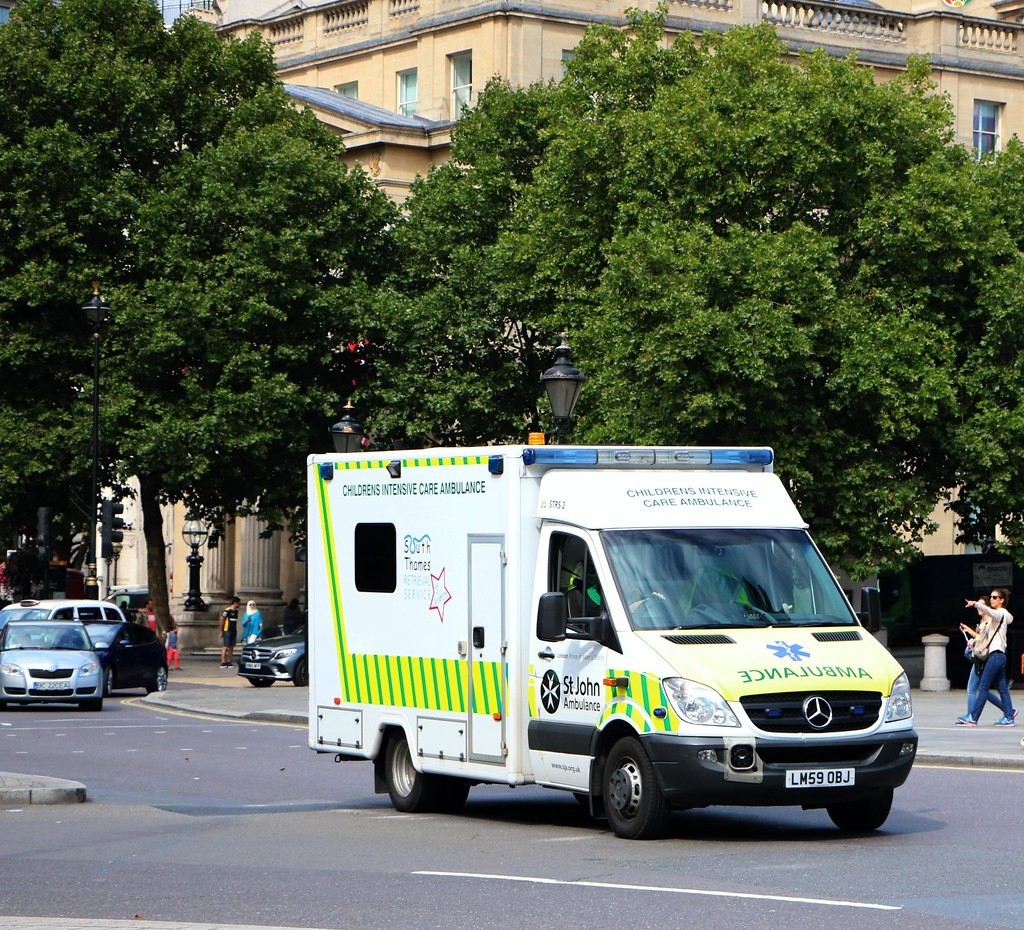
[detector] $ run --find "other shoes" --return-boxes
[168,669,173,671]
[174,667,183,671]
[226,662,234,668]
[220,663,227,669]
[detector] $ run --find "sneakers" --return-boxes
[956,721,964,726]
[994,717,1015,726]
[1012,709,1018,718]
[958,717,977,727]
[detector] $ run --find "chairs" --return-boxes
[566,586,600,634]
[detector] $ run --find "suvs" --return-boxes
[87,584,149,620]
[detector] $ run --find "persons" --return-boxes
[136,599,166,638]
[119,601,132,623]
[217,597,241,668]
[956,596,1019,726]
[241,600,263,644]
[958,588,1015,727]
[164,623,183,670]
[567,540,665,623]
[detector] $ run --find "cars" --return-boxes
[237,623,308,688]
[0,621,104,711]
[43,620,168,698]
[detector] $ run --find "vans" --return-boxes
[1,599,127,650]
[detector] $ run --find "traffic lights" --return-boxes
[102,500,123,558]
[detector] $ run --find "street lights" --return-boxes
[540,332,587,444]
[82,285,110,599]
[181,518,208,612]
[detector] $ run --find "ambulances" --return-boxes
[306,433,918,840]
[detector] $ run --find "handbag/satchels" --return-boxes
[974,643,988,660]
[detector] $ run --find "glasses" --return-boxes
[989,596,1000,599]
